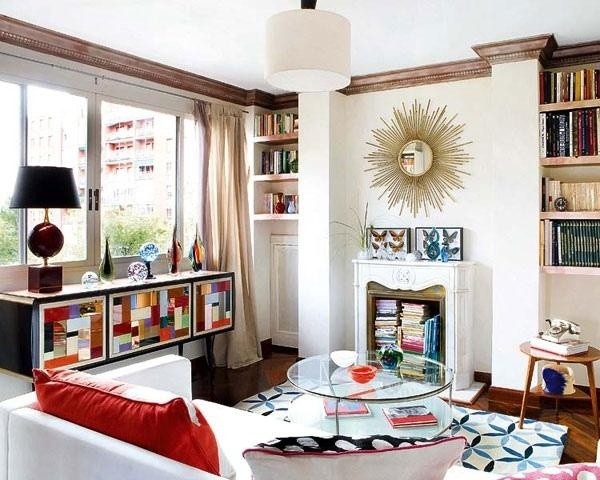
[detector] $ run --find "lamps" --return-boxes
[266,0,351,91]
[10,166,82,292]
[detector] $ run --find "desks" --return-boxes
[519,341,599,441]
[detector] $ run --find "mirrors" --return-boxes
[398,139,434,176]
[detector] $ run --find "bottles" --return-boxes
[97,236,116,286]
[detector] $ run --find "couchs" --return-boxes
[0,354,504,480]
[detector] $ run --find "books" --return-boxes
[537,66,600,269]
[381,403,438,429]
[372,299,439,385]
[530,334,588,356]
[254,110,298,175]
[263,192,298,214]
[322,395,371,418]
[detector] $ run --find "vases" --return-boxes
[328,202,385,261]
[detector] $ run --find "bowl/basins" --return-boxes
[331,350,358,368]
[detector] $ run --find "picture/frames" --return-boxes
[366,227,464,262]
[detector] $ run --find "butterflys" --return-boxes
[371,230,405,254]
[422,229,460,258]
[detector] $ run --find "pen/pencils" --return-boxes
[383,370,397,373]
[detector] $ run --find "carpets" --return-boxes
[231,379,571,475]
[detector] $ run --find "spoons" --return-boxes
[348,364,378,385]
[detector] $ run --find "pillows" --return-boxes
[243,434,466,480]
[31,366,235,480]
[496,463,599,480]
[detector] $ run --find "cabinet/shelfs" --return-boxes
[1,269,235,376]
[254,131,299,220]
[539,98,600,275]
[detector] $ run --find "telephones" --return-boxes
[539,318,581,343]
